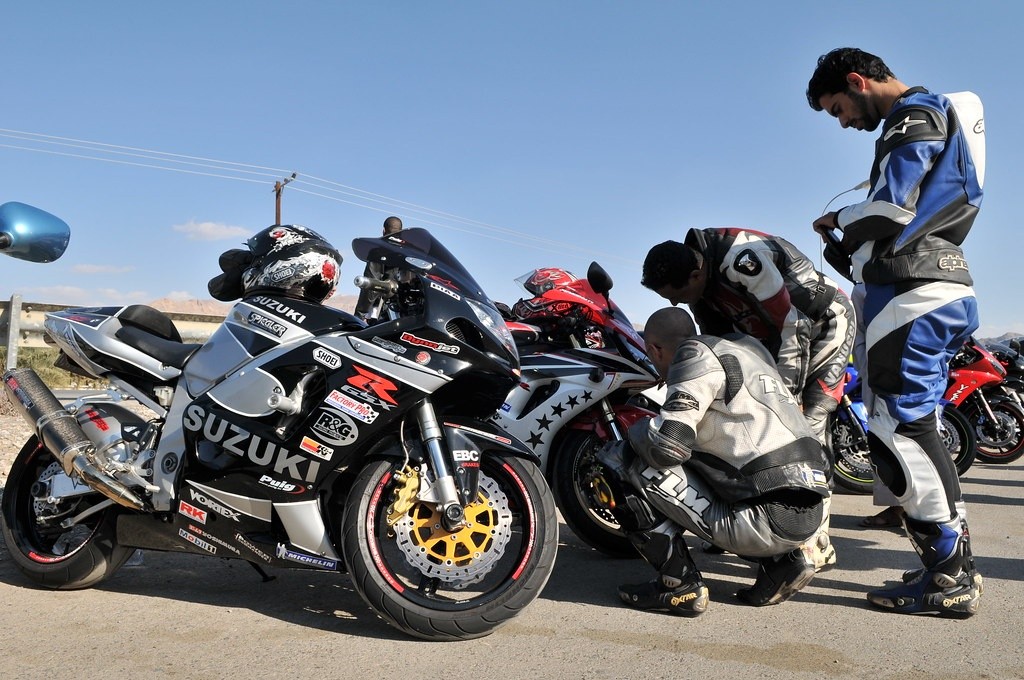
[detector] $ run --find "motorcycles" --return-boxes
[827,327,1024,494]
[1,225,561,645]
[349,218,685,561]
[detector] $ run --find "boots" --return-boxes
[701,541,727,554]
[616,517,709,617]
[867,512,976,618]
[736,548,809,606]
[801,490,837,573]
[902,499,984,597]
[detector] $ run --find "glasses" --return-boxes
[643,345,662,364]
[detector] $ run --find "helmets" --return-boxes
[511,268,579,321]
[241,224,343,305]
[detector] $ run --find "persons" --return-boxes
[353,217,403,323]
[599,47,986,616]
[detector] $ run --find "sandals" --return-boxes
[863,507,902,527]
